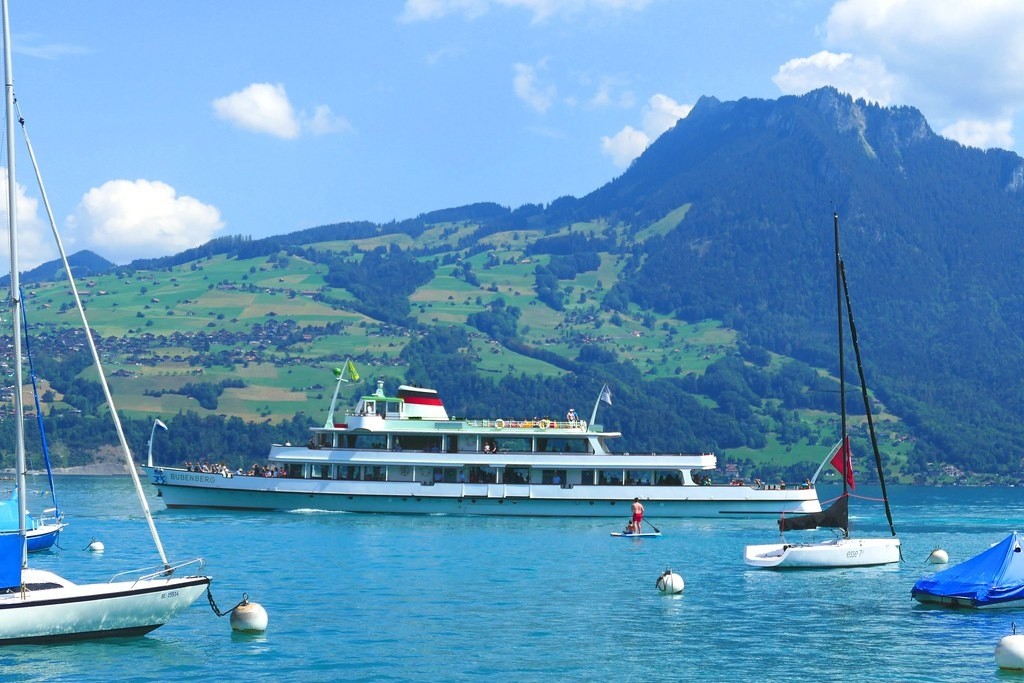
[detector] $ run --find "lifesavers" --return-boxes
[495,419,505,429]
[539,419,549,430]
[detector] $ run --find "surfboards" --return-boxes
[610,532,662,536]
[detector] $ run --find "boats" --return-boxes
[911,530,1024,614]
[742,213,904,572]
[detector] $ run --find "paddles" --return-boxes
[642,517,660,533]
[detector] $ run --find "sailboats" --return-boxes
[0,0,214,647]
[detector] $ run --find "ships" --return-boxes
[140,356,824,523]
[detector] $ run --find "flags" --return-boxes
[829,435,856,490]
[601,385,613,406]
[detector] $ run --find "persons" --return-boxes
[567,408,579,427]
[781,480,786,489]
[367,402,371,414]
[458,470,466,483]
[609,473,712,486]
[509,416,557,430]
[626,498,645,534]
[756,476,761,487]
[310,436,315,447]
[434,470,443,482]
[730,479,744,487]
[184,460,286,480]
[470,469,529,484]
[485,440,498,454]
[553,473,560,484]
